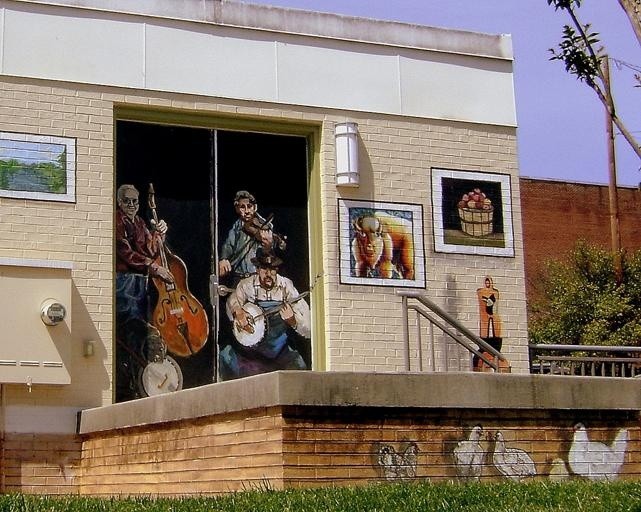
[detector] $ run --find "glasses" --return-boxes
[121,196,139,205]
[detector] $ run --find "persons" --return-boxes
[114,185,169,353]
[219,246,311,381]
[218,190,274,288]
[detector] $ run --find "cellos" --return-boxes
[148,183,210,358]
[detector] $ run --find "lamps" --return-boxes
[335,120,359,188]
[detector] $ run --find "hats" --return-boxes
[250,247,284,269]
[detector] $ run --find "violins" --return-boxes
[242,217,286,250]
